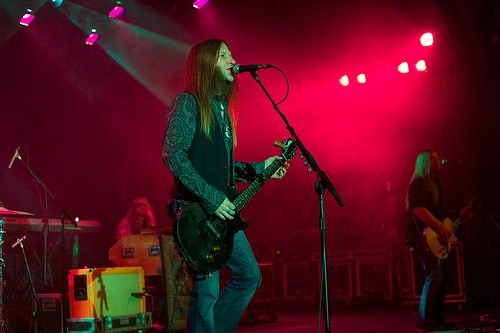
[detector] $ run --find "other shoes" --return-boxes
[415,319,464,332]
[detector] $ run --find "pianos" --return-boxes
[0,217,103,305]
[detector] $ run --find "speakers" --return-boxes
[407,241,468,312]
[67,267,146,319]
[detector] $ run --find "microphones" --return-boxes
[131,291,146,299]
[8,145,20,172]
[11,236,28,248]
[441,159,462,164]
[233,64,272,74]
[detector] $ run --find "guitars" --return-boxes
[423,197,478,259]
[173,137,298,275]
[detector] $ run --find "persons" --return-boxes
[115,197,156,240]
[162,38,291,333]
[406,150,473,328]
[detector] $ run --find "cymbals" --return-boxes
[0,206,36,216]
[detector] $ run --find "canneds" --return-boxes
[141,312,146,325]
[105,316,112,331]
[479,315,490,333]
